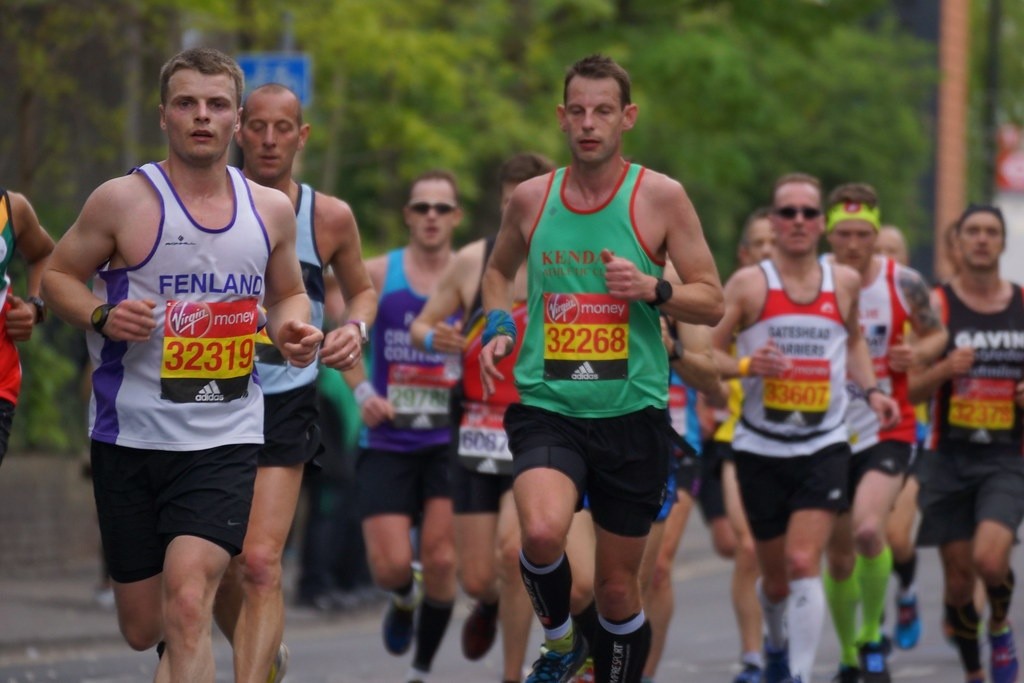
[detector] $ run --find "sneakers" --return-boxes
[988,617,1019,683]
[894,590,922,650]
[525,624,596,683]
[831,634,893,683]
[734,635,795,683]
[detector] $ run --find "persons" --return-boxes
[411,155,554,683]
[213,86,379,683]
[905,201,1024,683]
[326,176,462,683]
[713,176,900,683]
[0,185,55,465]
[40,51,324,683]
[566,254,720,683]
[479,56,725,683]
[823,182,949,683]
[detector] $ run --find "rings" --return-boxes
[349,354,355,359]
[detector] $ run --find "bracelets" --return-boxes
[423,328,437,354]
[863,386,885,406]
[354,382,375,405]
[739,356,750,377]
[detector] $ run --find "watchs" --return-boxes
[25,294,47,325]
[342,318,370,345]
[646,277,673,311]
[668,339,682,362]
[90,303,115,340]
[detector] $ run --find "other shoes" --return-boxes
[461,596,500,661]
[267,640,289,683]
[382,596,415,656]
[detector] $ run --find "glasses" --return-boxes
[407,200,458,215]
[774,206,823,220]
